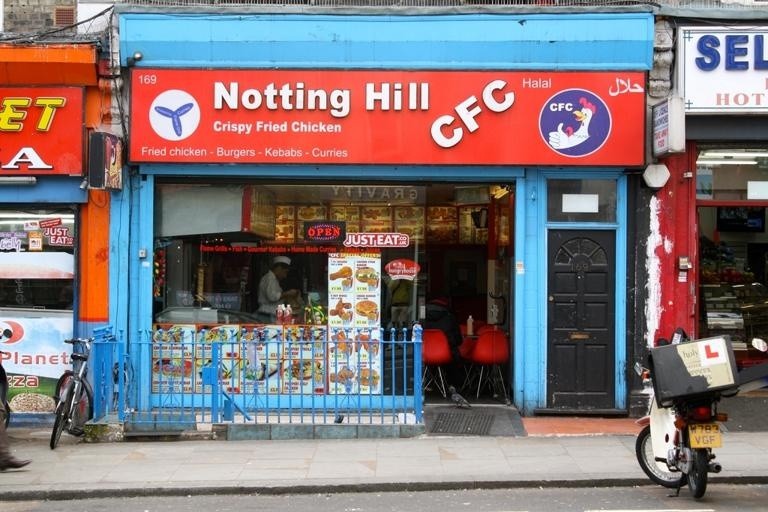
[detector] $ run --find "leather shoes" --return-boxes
[1,457,30,472]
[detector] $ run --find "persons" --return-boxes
[0,365,32,471]
[257,255,298,320]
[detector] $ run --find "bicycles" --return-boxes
[49,338,98,452]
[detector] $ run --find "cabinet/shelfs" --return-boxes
[698,282,768,386]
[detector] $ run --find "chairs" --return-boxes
[420,321,512,407]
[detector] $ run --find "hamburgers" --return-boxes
[357,368,378,385]
[356,267,375,282]
[356,301,377,316]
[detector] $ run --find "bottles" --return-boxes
[275,304,292,325]
[466,315,475,337]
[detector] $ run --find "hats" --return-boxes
[272,256,291,265]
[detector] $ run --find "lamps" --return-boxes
[696,151,768,166]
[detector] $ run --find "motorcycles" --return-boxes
[635,327,739,498]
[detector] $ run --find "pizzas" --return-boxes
[10,393,56,412]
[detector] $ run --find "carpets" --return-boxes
[429,411,495,436]
[715,396,768,432]
